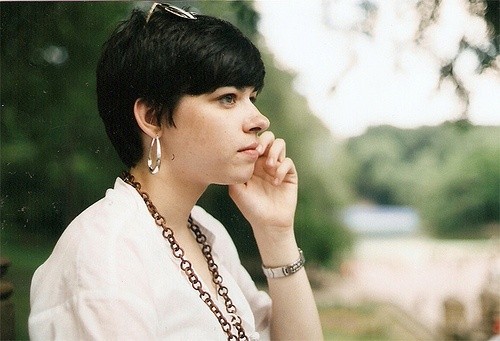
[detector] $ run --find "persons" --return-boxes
[28,2,323,341]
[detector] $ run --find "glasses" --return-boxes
[142,3,198,31]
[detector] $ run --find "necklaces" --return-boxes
[122,171,247,341]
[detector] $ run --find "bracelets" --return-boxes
[260,246,304,280]
